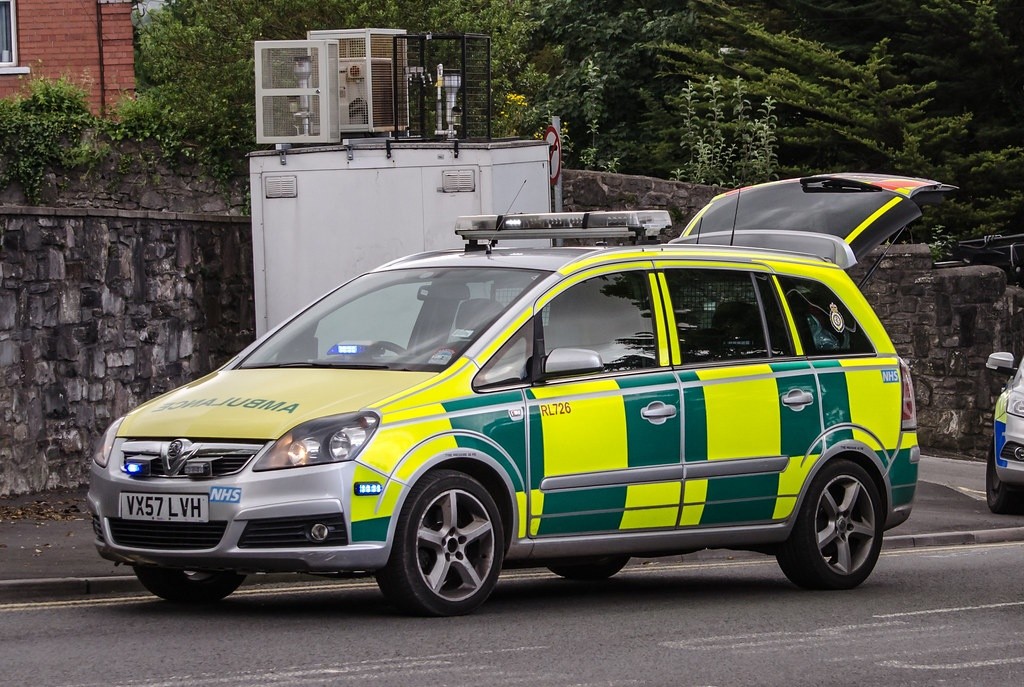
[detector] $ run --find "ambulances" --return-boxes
[80,170,960,618]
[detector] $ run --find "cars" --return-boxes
[979,347,1023,514]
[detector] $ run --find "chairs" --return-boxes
[451,299,505,350]
[710,300,761,355]
